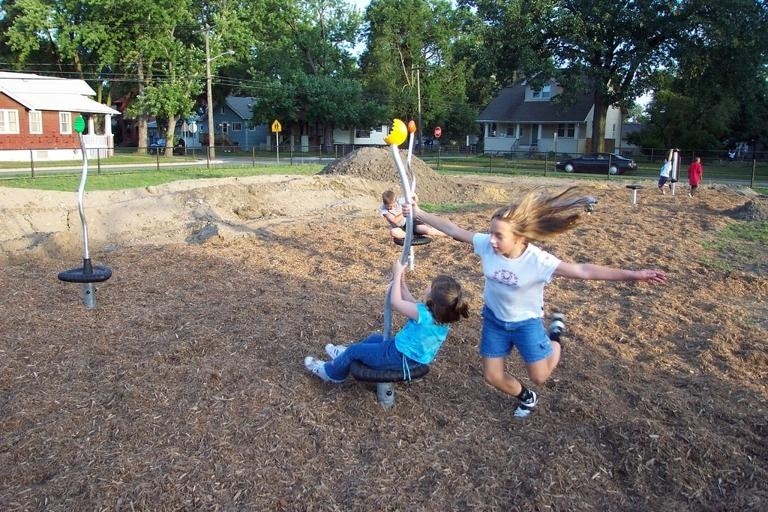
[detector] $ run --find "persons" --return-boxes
[300,251,465,388]
[379,190,451,240]
[687,156,702,199]
[657,148,675,195]
[403,185,668,419]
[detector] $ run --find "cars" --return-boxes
[148,137,187,155]
[555,153,638,175]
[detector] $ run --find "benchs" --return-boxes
[149,146,184,155]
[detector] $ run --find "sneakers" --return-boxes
[325,343,349,360]
[303,356,346,383]
[546,311,565,335]
[513,390,539,420]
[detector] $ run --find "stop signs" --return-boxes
[434,127,441,138]
